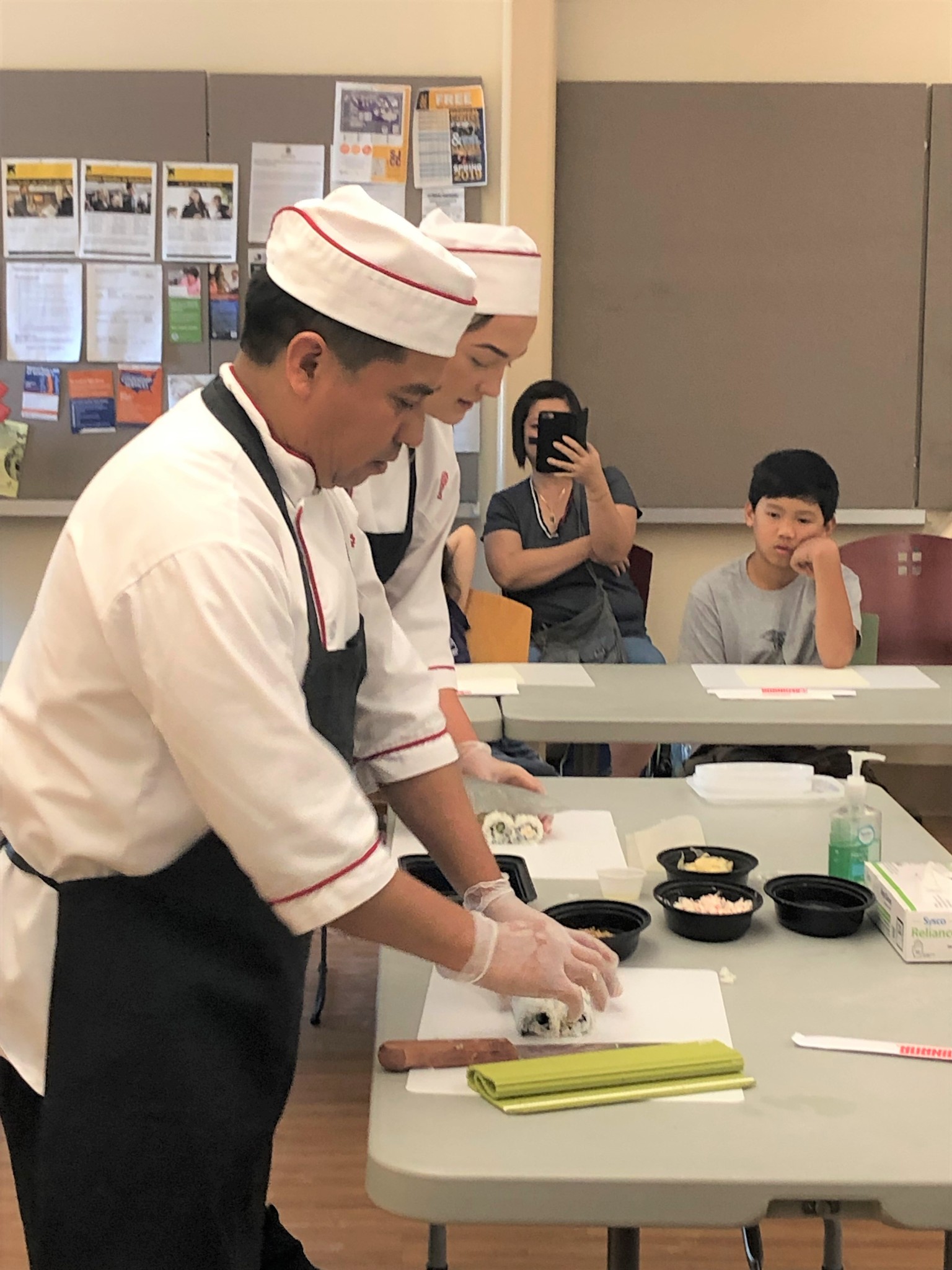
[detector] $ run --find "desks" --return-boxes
[310,660,951,1270]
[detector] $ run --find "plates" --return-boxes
[685,773,846,806]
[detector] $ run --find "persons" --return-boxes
[439,521,563,780]
[168,186,232,220]
[83,179,153,215]
[2,179,629,1268]
[324,202,545,821]
[680,450,865,787]
[8,181,74,218]
[483,378,689,781]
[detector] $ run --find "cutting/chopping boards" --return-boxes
[406,966,745,1105]
[389,808,627,880]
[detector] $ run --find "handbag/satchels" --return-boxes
[531,588,627,664]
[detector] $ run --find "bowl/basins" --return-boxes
[538,899,652,962]
[656,844,760,886]
[763,873,876,939]
[398,854,538,903]
[652,877,764,943]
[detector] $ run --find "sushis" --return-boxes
[481,811,544,846]
[511,986,590,1037]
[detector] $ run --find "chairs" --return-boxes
[463,544,654,659]
[837,533,952,667]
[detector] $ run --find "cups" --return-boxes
[597,867,647,905]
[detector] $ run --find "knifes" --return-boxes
[377,1037,676,1073]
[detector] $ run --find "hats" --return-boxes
[266,185,477,358]
[415,210,540,318]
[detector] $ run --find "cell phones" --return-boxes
[537,411,574,473]
